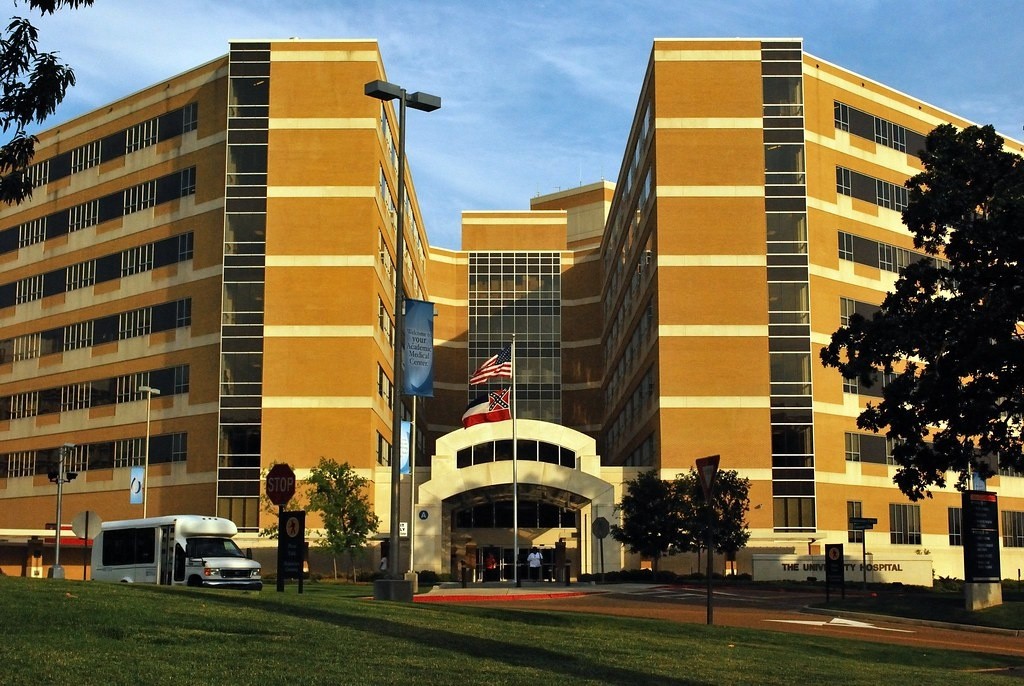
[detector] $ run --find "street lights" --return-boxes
[364,78,441,608]
[138,385,161,519]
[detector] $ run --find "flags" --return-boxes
[461,388,512,430]
[469,343,513,386]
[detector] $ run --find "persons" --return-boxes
[527,546,543,583]
[379,555,388,572]
[486,554,497,582]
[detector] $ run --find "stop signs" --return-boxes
[265,463,296,506]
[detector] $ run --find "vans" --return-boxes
[90,514,263,591]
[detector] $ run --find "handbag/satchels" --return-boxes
[540,559,543,565]
